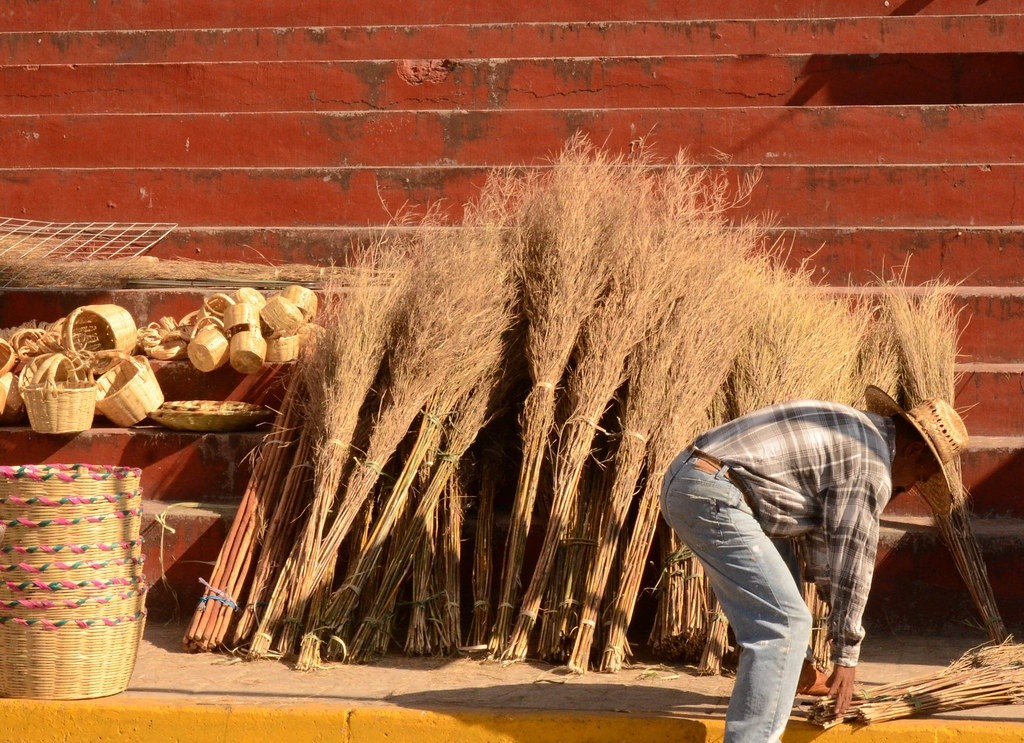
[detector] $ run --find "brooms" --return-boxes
[0,125,1024,732]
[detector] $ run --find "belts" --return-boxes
[688,444,762,521]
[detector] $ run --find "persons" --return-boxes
[659,395,969,743]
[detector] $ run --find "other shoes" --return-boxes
[794,661,831,706]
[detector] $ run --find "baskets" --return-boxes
[0,464,147,699]
[138,287,327,372]
[0,304,164,434]
[146,400,273,431]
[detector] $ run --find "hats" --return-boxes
[864,384,970,516]
